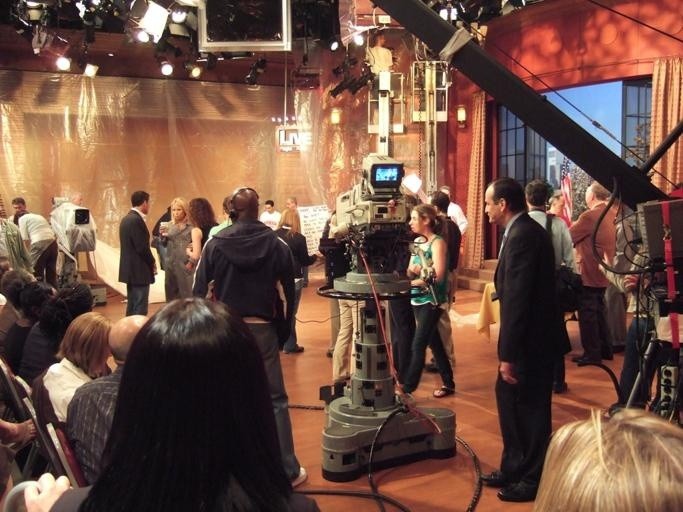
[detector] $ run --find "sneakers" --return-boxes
[285,346,304,354]
[292,468,308,489]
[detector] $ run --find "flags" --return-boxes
[560,156,573,226]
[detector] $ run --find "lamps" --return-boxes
[18,0,202,81]
[456,102,466,129]
[241,54,267,90]
[290,14,377,99]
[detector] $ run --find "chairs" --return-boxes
[0,355,84,489]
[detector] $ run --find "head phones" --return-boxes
[229,186,259,220]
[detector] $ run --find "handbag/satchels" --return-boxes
[556,264,582,312]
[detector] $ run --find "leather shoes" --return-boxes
[480,470,510,486]
[498,483,537,503]
[394,343,628,397]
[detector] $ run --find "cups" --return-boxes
[161,222,172,236]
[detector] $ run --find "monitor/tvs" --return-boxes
[198,0,292,51]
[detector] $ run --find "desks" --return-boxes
[475,281,575,344]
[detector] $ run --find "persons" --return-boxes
[118,191,158,317]
[318,186,468,414]
[524,181,578,394]
[534,407,678,506]
[151,197,233,303]
[1,197,113,506]
[260,199,283,231]
[598,244,678,424]
[547,182,633,367]
[66,315,151,484]
[192,187,307,487]
[274,208,323,353]
[77,298,318,507]
[285,197,309,287]
[480,177,555,502]
